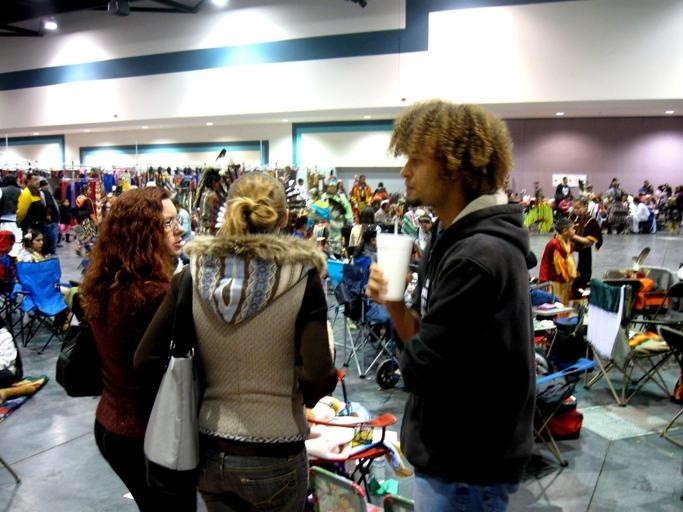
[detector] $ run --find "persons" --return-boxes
[507,177,683,235]
[201,169,224,236]
[131,168,339,512]
[364,97,537,511]
[0,173,99,288]
[173,199,192,240]
[281,173,434,273]
[76,184,201,512]
[539,199,602,308]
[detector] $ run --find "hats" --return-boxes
[40,180,48,187]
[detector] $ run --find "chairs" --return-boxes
[0,241,683,512]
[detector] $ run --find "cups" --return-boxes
[375,233,414,302]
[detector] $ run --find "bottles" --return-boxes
[372,456,385,483]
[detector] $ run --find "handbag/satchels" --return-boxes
[56,325,103,397]
[144,348,200,471]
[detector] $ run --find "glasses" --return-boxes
[162,215,181,232]
